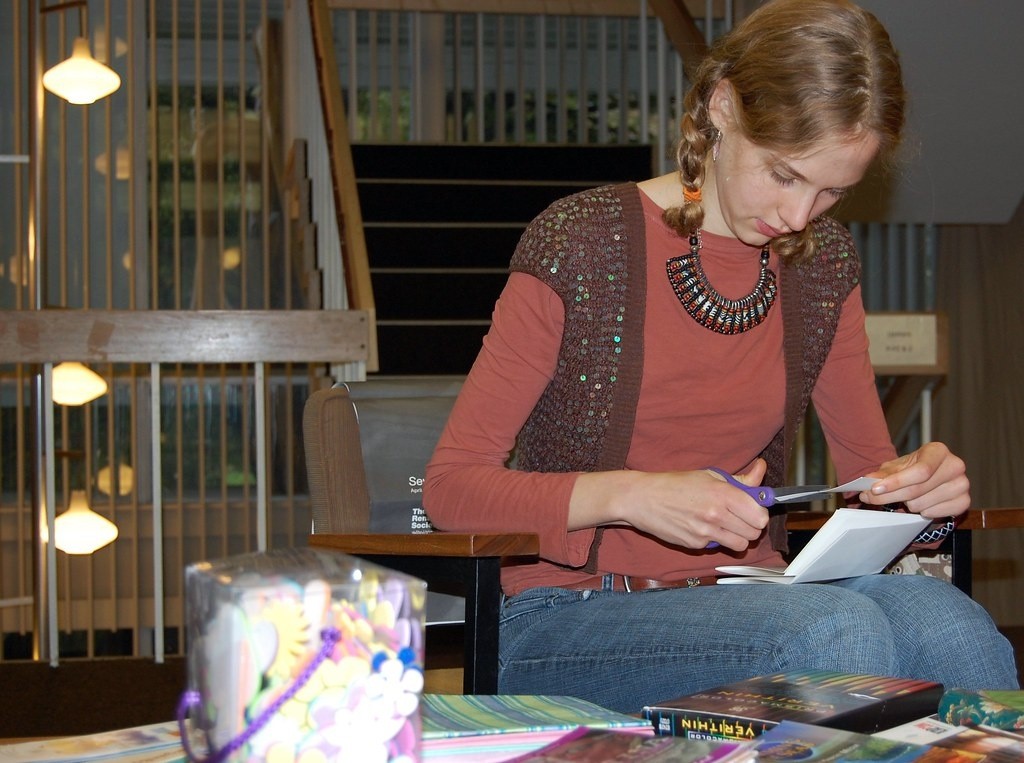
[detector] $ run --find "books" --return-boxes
[0,666,1024,763]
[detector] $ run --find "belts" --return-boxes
[561,573,718,592]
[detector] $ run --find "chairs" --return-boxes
[295,374,1024,698]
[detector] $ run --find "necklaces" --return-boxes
[666,174,777,335]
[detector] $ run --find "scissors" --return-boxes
[701,467,834,548]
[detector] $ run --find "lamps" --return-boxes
[42,37,121,104]
[97,464,133,496]
[50,362,108,405]
[41,490,119,556]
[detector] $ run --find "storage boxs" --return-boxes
[642,668,945,741]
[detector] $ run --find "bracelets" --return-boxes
[884,505,955,544]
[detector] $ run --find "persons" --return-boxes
[420,0,1024,713]
